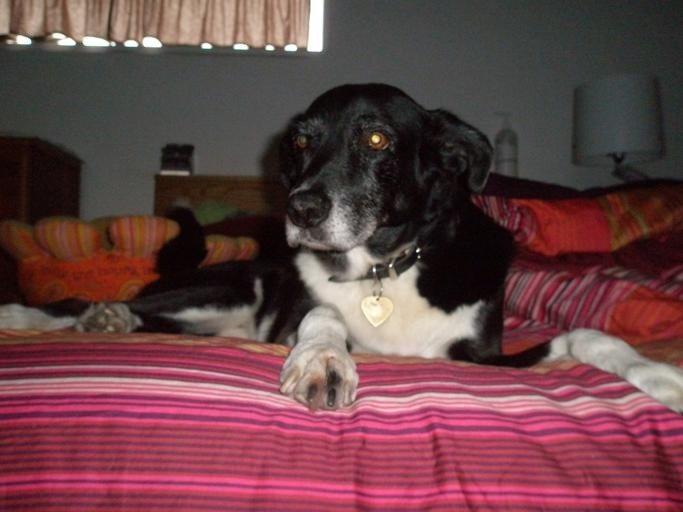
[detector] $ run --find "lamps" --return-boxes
[573,73,663,184]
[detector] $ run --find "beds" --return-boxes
[0,178,683,509]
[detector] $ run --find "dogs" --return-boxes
[0,82,683,418]
